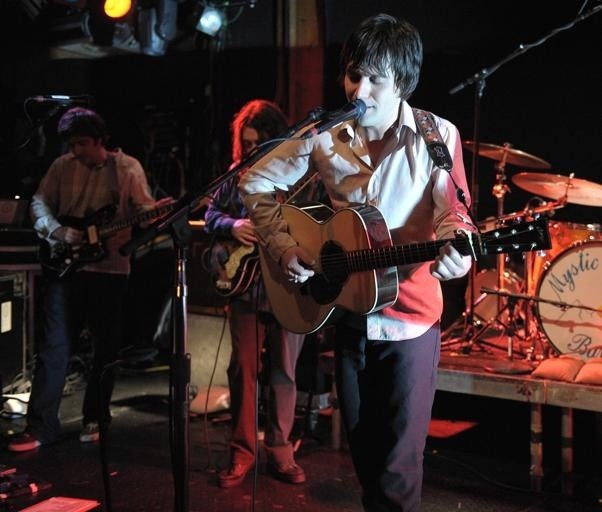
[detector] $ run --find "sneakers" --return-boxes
[266,459,306,484]
[9,430,41,452]
[79,423,111,442]
[217,457,256,487]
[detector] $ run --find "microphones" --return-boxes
[300,99,366,141]
[34,91,92,107]
[257,103,324,161]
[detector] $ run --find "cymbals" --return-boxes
[512,169,602,211]
[463,136,549,170]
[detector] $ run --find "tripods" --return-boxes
[462,253,548,360]
[437,172,528,361]
[288,336,329,456]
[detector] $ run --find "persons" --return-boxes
[201,101,307,487]
[10,108,174,451]
[238,17,478,508]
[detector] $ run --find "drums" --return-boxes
[464,267,526,328]
[534,237,602,363]
[527,220,600,298]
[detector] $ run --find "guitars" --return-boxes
[39,193,186,273]
[205,178,317,298]
[250,196,552,338]
